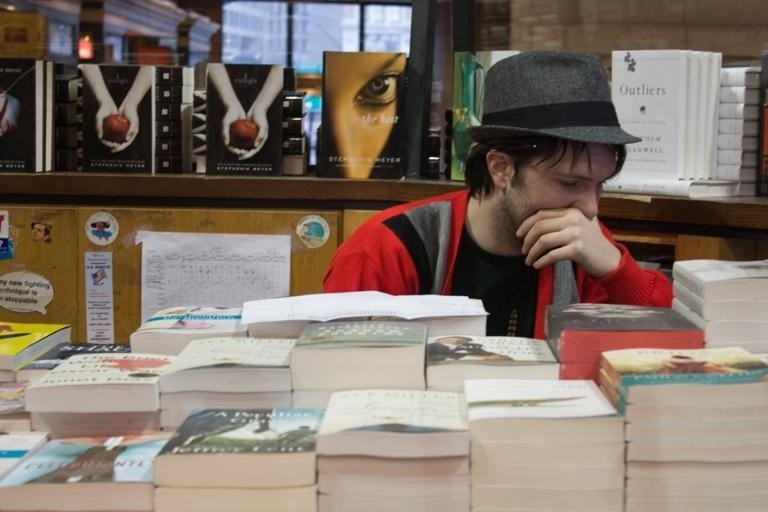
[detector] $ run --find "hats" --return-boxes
[465,50,642,144]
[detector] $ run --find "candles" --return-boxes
[77,34,95,63]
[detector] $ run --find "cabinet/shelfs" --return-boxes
[0,203,341,346]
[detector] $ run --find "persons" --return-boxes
[321,49,673,337]
[31,223,51,242]
[326,51,406,180]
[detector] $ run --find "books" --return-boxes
[600,47,761,199]
[1,306,319,512]
[0,55,309,176]
[246,259,768,512]
[450,48,518,185]
